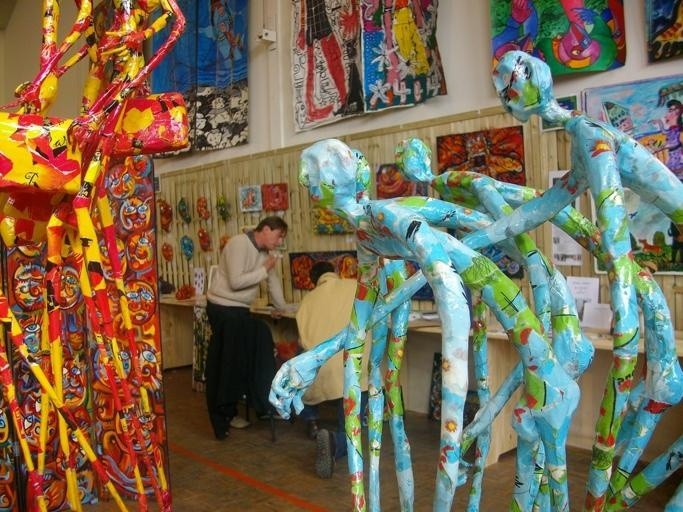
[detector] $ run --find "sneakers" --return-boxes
[308,419,319,440]
[230,416,250,428]
[314,428,336,479]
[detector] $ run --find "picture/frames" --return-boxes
[581,71,682,276]
[537,92,581,132]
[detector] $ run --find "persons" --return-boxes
[192,216,286,430]
[292,260,363,479]
